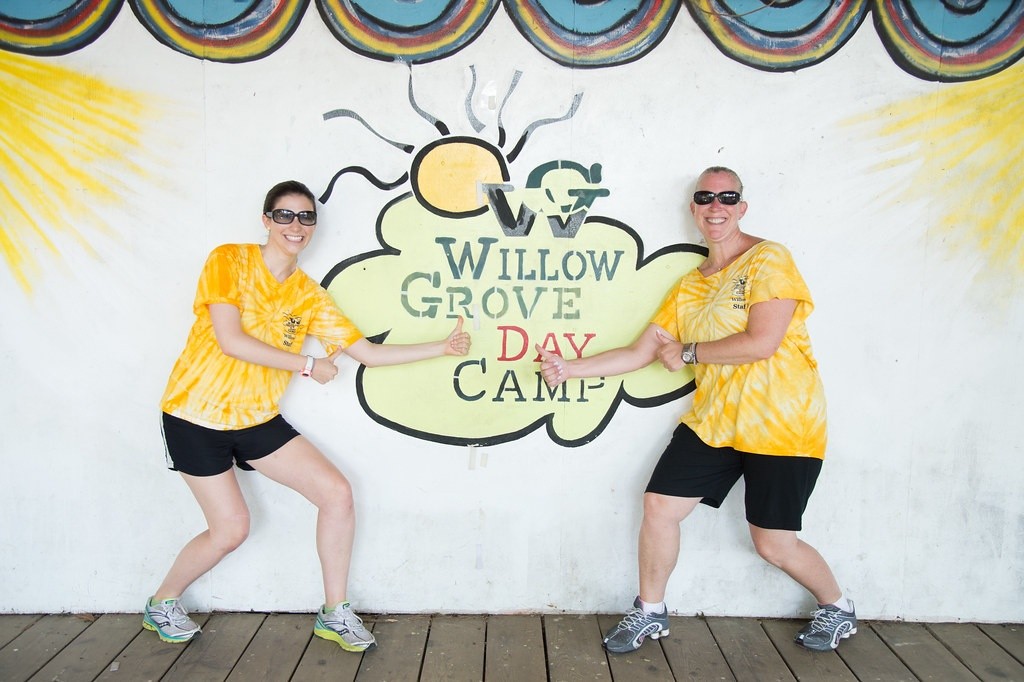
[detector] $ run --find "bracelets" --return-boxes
[693,341,699,363]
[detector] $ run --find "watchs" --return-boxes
[681,343,695,365]
[300,355,314,377]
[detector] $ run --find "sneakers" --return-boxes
[793,598,858,651]
[142,596,202,644]
[601,596,671,653]
[313,603,378,653]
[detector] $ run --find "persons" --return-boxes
[142,179,473,653]
[534,165,858,654]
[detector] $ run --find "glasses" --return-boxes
[265,209,318,227]
[692,190,744,205]
